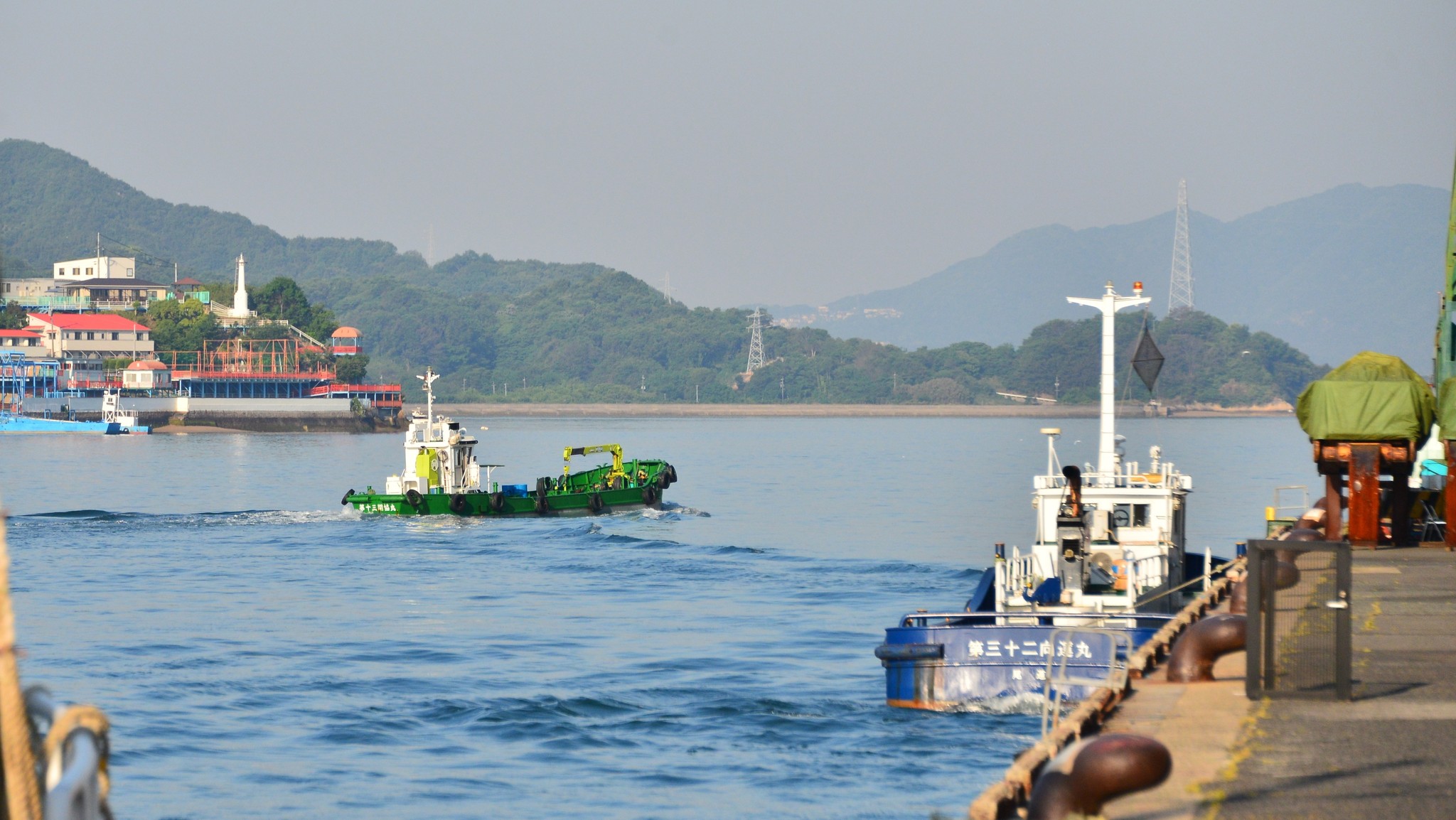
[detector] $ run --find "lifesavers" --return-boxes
[1131,473,1162,483]
[449,493,467,513]
[536,496,550,514]
[642,486,657,505]
[341,488,355,506]
[588,494,603,513]
[490,492,504,512]
[658,471,671,489]
[124,428,128,433]
[668,465,677,483]
[406,489,423,506]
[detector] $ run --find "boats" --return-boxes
[0,349,152,434]
[877,280,1234,720]
[341,365,679,520]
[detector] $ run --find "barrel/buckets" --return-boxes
[502,484,528,498]
